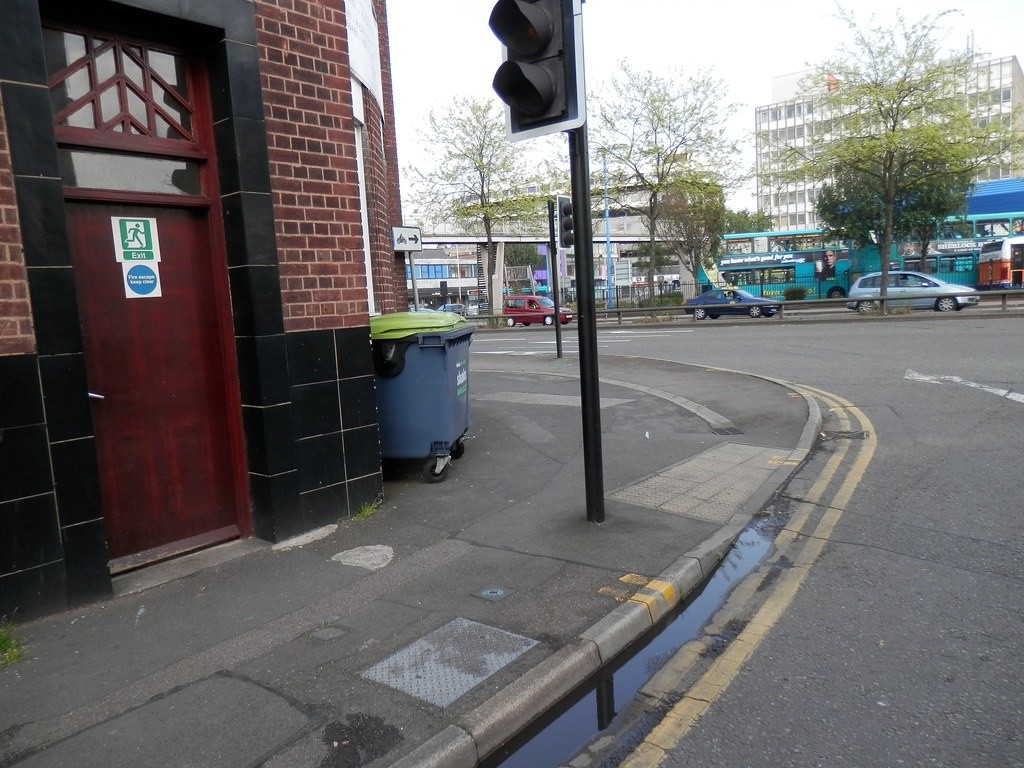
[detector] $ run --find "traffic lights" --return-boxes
[558,196,573,248]
[490,1,585,142]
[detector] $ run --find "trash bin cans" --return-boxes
[369,311,477,482]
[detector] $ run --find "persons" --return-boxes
[902,243,919,256]
[816,250,835,280]
[530,301,537,309]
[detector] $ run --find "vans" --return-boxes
[503,296,574,326]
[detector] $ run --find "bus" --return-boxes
[505,286,551,299]
[698,212,1024,295]
[978,237,1024,291]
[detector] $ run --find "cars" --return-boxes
[685,288,783,321]
[846,270,980,312]
[411,307,436,312]
[438,304,469,316]
